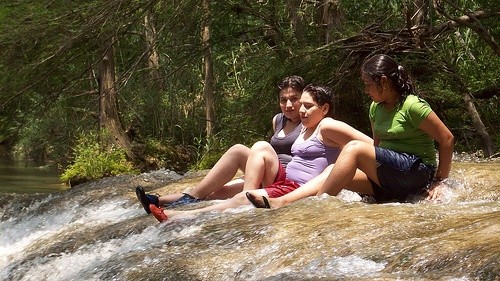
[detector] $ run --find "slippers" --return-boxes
[246,192,270,210]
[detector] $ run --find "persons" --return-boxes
[147,83,374,225]
[245,53,454,215]
[135,76,309,216]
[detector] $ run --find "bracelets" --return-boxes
[436,176,449,182]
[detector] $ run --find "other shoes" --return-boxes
[149,204,168,223]
[169,194,200,207]
[136,186,159,215]
